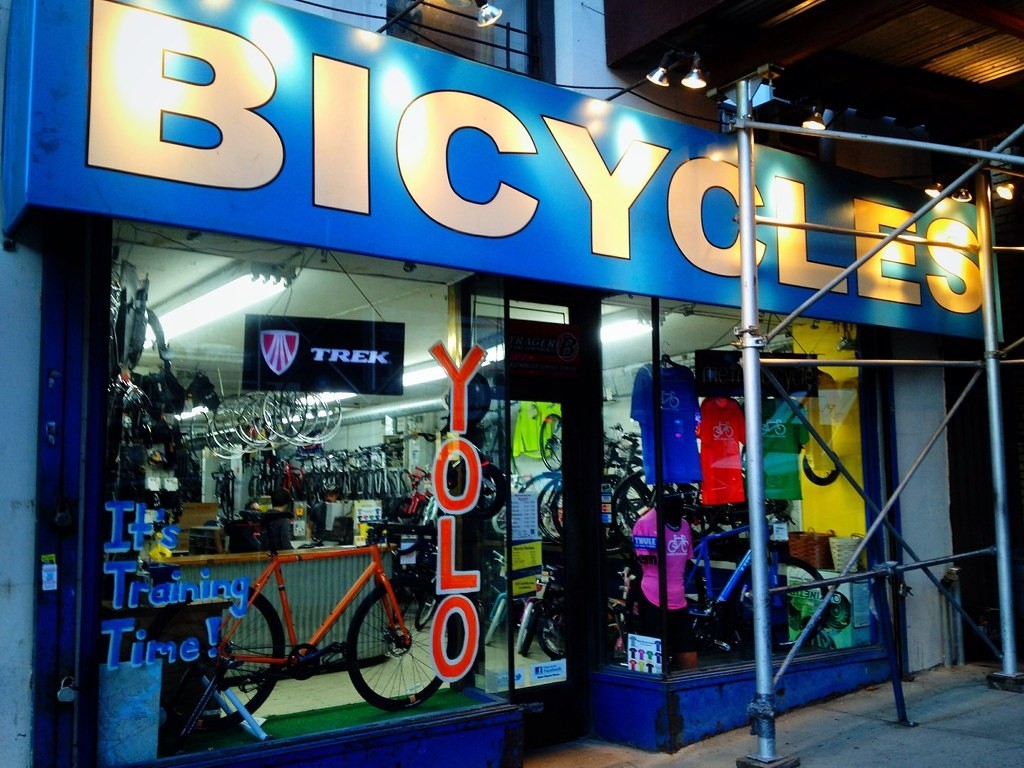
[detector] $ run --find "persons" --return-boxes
[632,497,697,667]
[306,483,342,543]
[228,489,295,554]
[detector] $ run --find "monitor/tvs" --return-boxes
[241,314,405,395]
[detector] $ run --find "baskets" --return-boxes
[788,531,836,570]
[829,537,863,572]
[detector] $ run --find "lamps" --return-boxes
[140,259,298,350]
[376,0,503,36]
[951,180,973,203]
[879,173,945,198]
[995,183,1017,200]
[765,101,827,130]
[604,50,708,101]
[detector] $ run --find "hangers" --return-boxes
[658,340,681,367]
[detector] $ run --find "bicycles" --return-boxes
[141,511,459,753]
[212,463,237,520]
[240,411,834,661]
